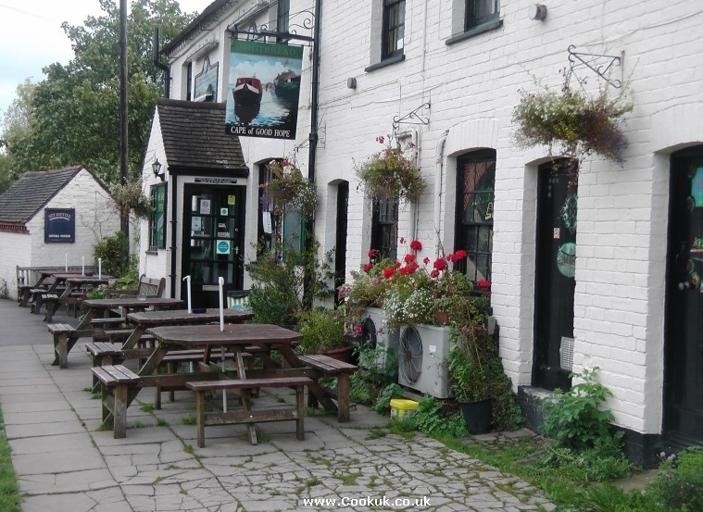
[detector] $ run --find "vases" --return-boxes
[457,394,493,435]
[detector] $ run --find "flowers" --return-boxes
[349,131,428,205]
[450,321,492,399]
[356,239,491,317]
[507,65,634,166]
[257,156,319,220]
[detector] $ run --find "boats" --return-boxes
[232,77,264,105]
[273,69,300,97]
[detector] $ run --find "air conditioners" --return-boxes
[354,305,460,400]
[151,158,165,181]
